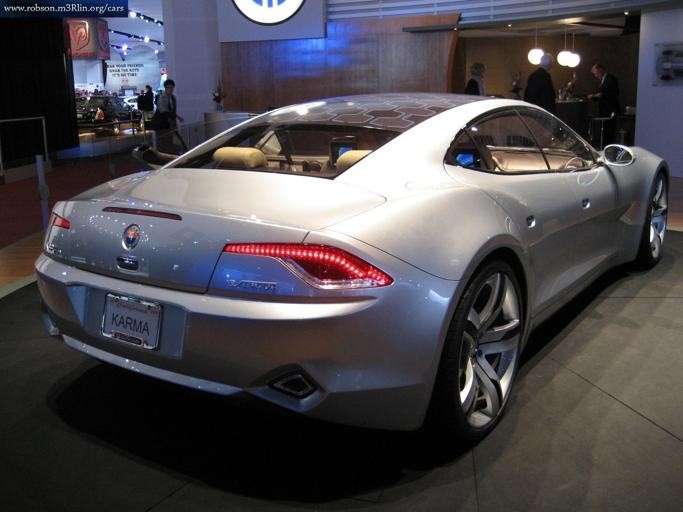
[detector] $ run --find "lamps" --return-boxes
[526,22,581,70]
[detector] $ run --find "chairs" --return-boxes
[211,144,266,170]
[337,149,371,171]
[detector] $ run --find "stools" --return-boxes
[588,114,611,151]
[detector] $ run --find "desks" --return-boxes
[555,96,585,138]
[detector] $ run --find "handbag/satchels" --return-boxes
[149,109,170,130]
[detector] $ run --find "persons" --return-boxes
[524,53,557,114]
[585,62,620,145]
[142,85,154,122]
[152,80,183,130]
[464,62,486,96]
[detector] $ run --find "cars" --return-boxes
[75,96,141,135]
[124,96,157,120]
[32,88,669,448]
